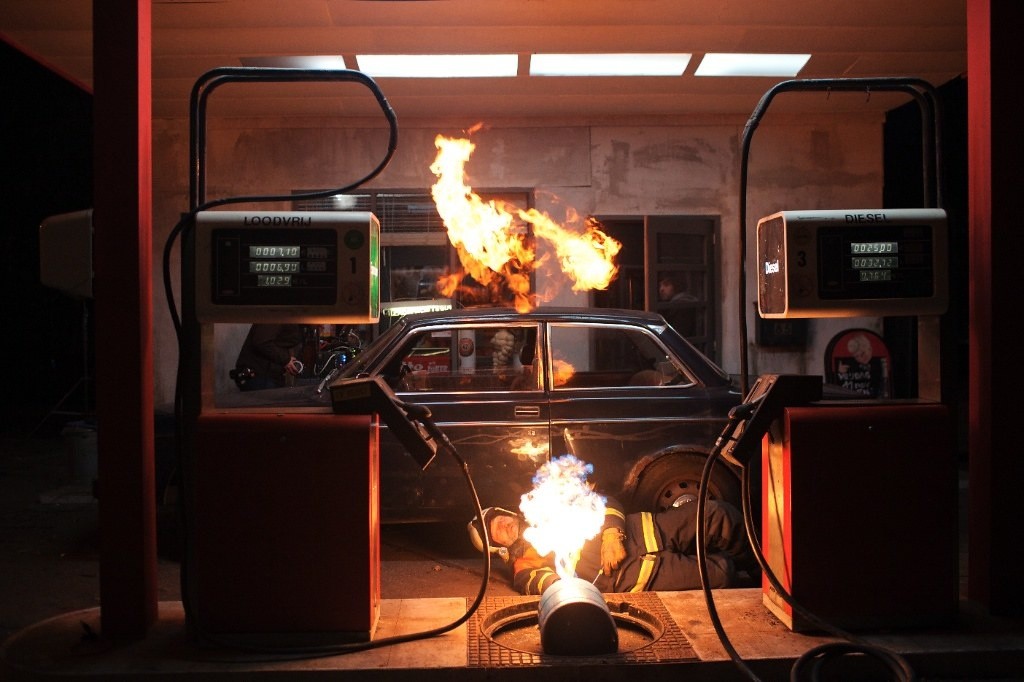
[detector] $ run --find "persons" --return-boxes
[469,498,742,596]
[230,323,308,394]
[653,273,703,338]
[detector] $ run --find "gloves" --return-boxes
[600,532,628,577]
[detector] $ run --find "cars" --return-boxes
[146,303,753,549]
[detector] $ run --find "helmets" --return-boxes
[467,506,523,563]
